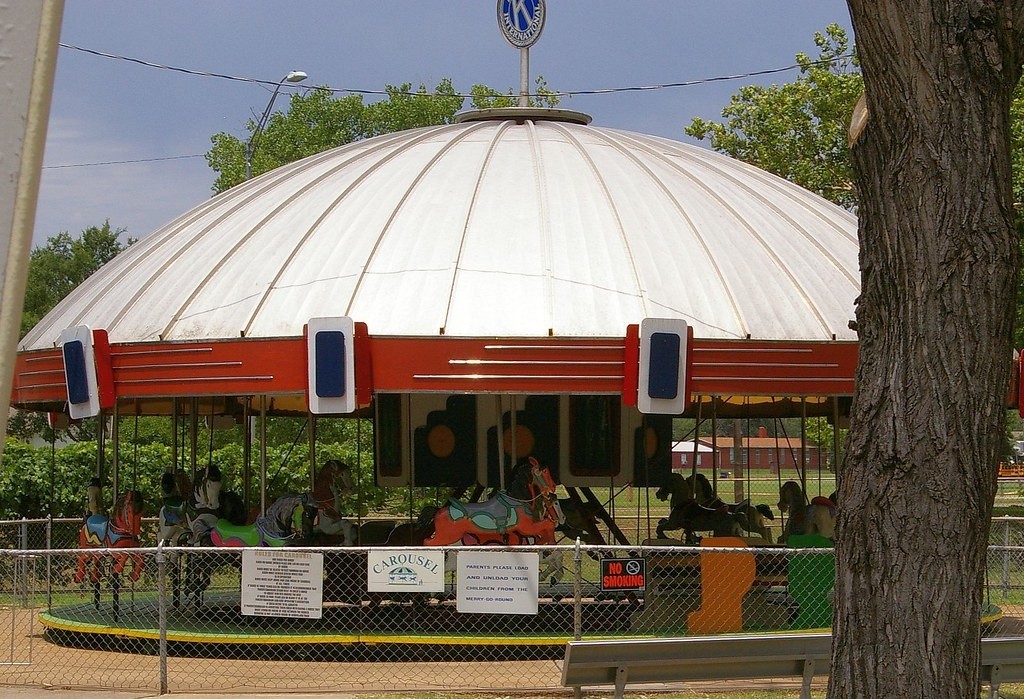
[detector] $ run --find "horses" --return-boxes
[416,457,558,586]
[552,496,615,559]
[184,493,310,596]
[302,459,357,547]
[156,463,247,568]
[777,480,838,544]
[655,474,744,542]
[686,472,774,544]
[442,492,566,581]
[72,489,146,585]
[82,478,110,523]
